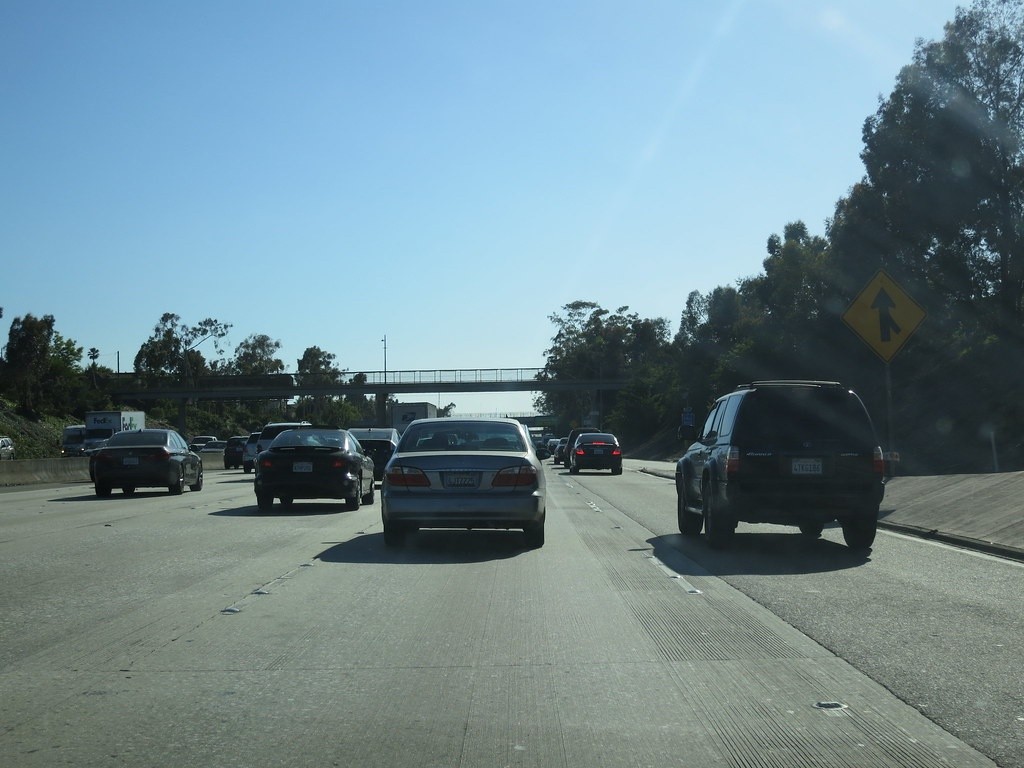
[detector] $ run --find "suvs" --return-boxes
[674,377,886,552]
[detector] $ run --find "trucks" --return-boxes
[81,410,145,457]
[60,424,85,458]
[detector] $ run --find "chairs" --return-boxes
[484,438,509,447]
[423,439,445,449]
[324,439,342,446]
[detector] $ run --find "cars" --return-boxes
[92,428,203,497]
[381,414,546,549]
[191,419,623,485]
[255,426,376,510]
[0,434,17,460]
